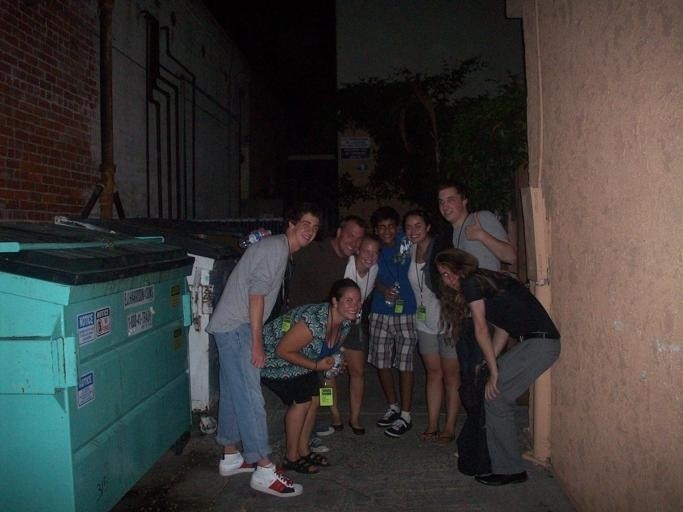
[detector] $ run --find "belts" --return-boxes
[520,329,560,338]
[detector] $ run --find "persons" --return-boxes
[429,248,562,486]
[400,210,461,449]
[259,276,361,475]
[366,206,418,439]
[436,181,518,459]
[281,215,366,310]
[201,199,322,498]
[322,234,380,437]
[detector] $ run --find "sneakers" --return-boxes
[218,451,255,476]
[311,438,330,454]
[250,462,303,498]
[376,408,412,438]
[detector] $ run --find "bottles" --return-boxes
[384,280,400,307]
[240,228,271,246]
[324,346,346,379]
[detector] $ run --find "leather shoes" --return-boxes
[348,419,366,436]
[329,424,345,432]
[475,470,530,487]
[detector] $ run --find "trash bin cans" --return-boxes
[0,216,243,512]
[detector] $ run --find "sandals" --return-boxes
[281,452,330,475]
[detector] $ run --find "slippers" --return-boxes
[416,430,456,445]
